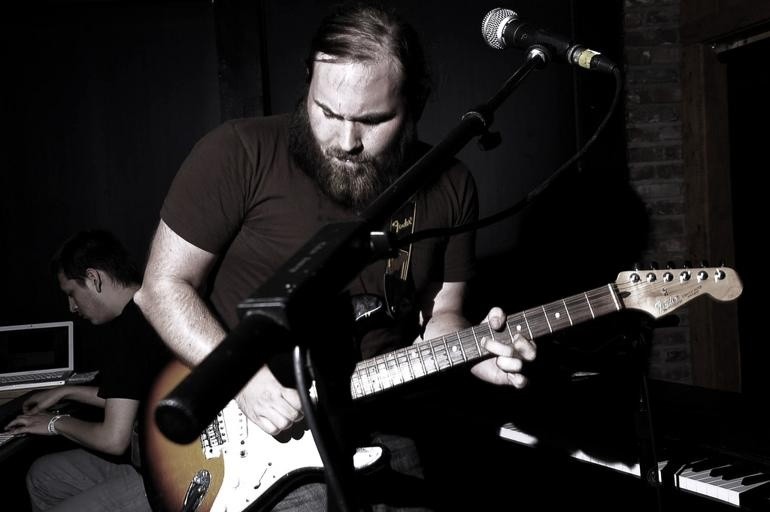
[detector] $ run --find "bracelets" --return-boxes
[47,413,72,435]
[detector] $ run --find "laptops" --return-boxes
[0,321,74,391]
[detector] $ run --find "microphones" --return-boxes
[481,7,618,75]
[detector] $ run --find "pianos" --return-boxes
[0,389,84,462]
[492,394,768,510]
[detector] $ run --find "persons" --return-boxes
[131,1,539,512]
[3,226,177,511]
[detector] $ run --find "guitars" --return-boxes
[136,260,743,512]
